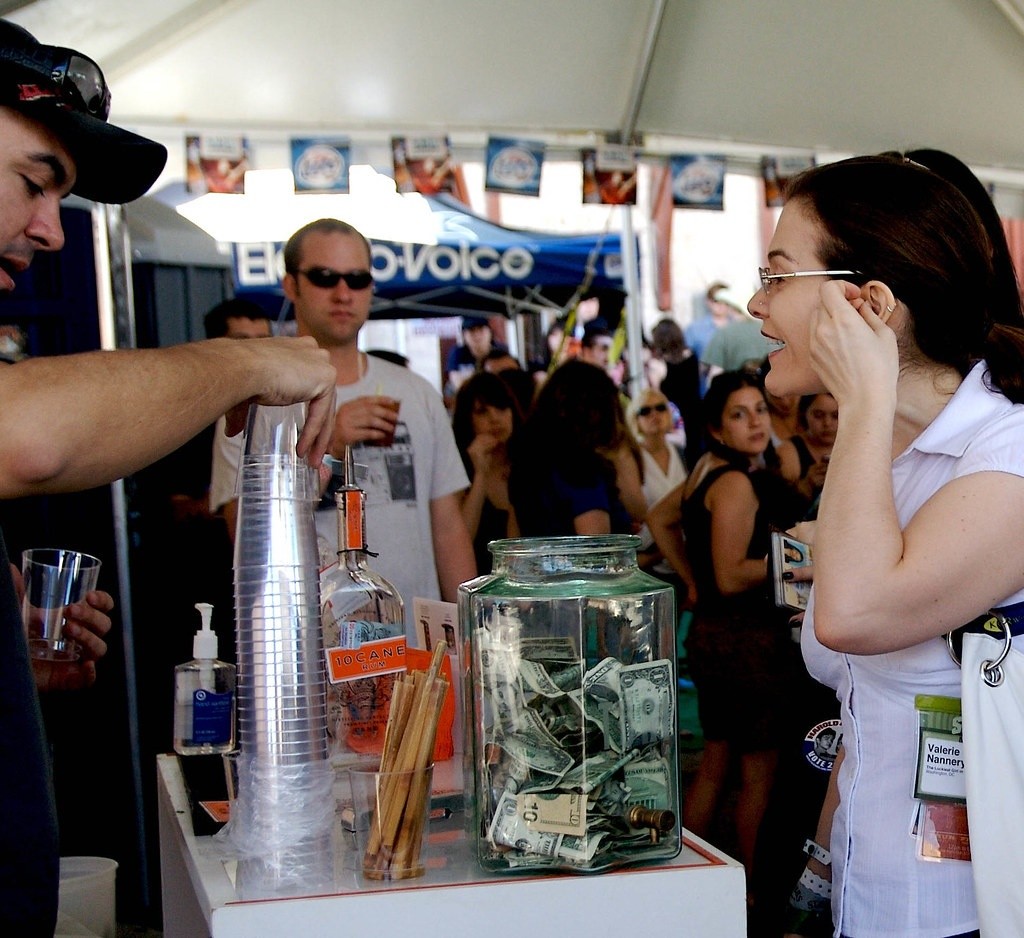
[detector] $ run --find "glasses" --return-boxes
[875,150,991,255]
[296,266,374,292]
[759,267,863,295]
[0,46,112,120]
[634,402,668,420]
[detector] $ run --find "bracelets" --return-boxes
[785,866,832,933]
[320,453,335,481]
[802,839,832,866]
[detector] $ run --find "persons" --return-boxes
[0,17,339,938]
[445,317,839,864]
[747,149,1024,938]
[172,297,272,519]
[208,218,478,647]
[686,283,783,389]
[783,539,804,564]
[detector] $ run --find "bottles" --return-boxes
[317,441,405,757]
[456,535,686,873]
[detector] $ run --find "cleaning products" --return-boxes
[173,602,238,756]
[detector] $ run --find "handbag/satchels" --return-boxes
[946,609,1024,938]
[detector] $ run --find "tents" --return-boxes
[176,192,627,331]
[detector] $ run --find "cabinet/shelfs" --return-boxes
[156,742,749,938]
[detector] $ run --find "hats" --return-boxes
[0,17,168,204]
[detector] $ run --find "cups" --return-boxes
[18,548,103,662]
[348,762,437,880]
[233,385,334,896]
